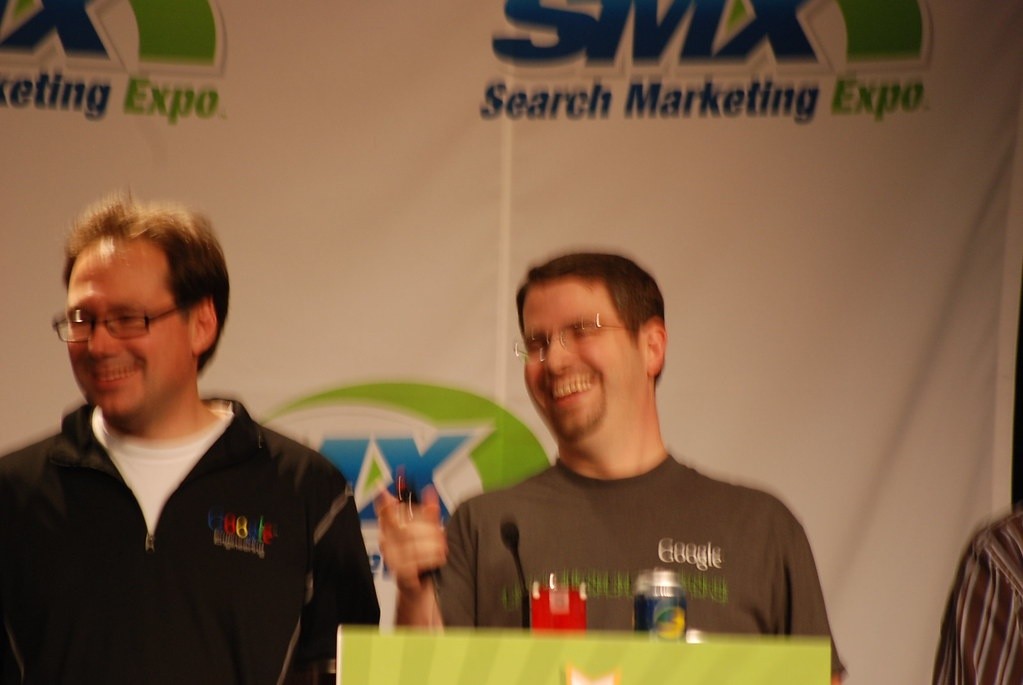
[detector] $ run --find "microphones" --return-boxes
[498,520,532,629]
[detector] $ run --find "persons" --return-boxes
[0,196,382,685]
[930,504,1023,685]
[378,252,845,685]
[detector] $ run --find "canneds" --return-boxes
[632,570,687,641]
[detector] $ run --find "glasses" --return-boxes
[52,303,184,342]
[514,314,628,361]
